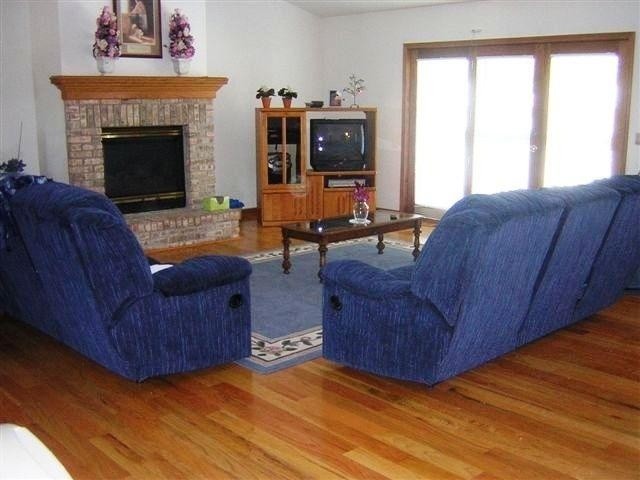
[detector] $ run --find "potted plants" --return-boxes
[256,88,298,109]
[349,180,371,223]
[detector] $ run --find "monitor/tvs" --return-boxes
[309,118,372,172]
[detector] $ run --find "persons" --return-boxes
[127,0,149,35]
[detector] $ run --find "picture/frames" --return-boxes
[113,0,163,59]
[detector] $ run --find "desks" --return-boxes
[278,206,425,277]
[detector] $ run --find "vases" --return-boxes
[95,53,194,76]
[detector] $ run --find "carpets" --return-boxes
[184,230,425,377]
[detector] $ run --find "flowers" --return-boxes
[92,6,196,60]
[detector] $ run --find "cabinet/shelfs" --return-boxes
[255,107,377,227]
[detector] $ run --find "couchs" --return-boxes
[320,173,636,387]
[2,172,257,386]
